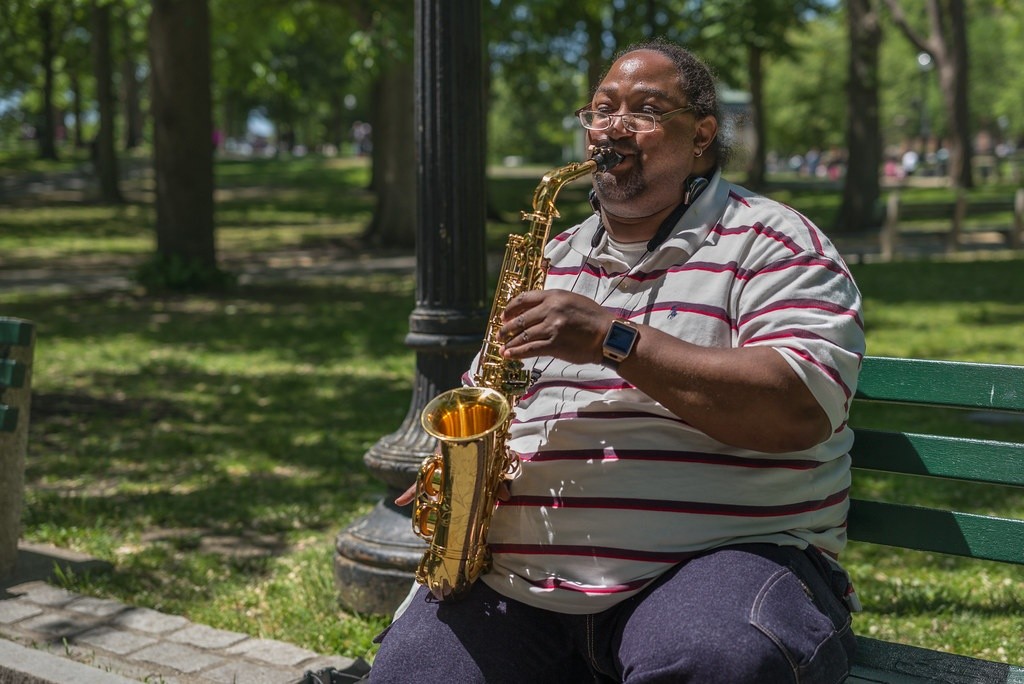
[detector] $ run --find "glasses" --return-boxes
[575,102,706,133]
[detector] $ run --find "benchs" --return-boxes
[834,355,1024,684]
[0,316,38,585]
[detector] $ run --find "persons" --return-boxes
[764,131,1012,179]
[357,41,862,684]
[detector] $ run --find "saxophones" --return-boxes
[412,144,626,604]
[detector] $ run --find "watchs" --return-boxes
[600,318,639,370]
